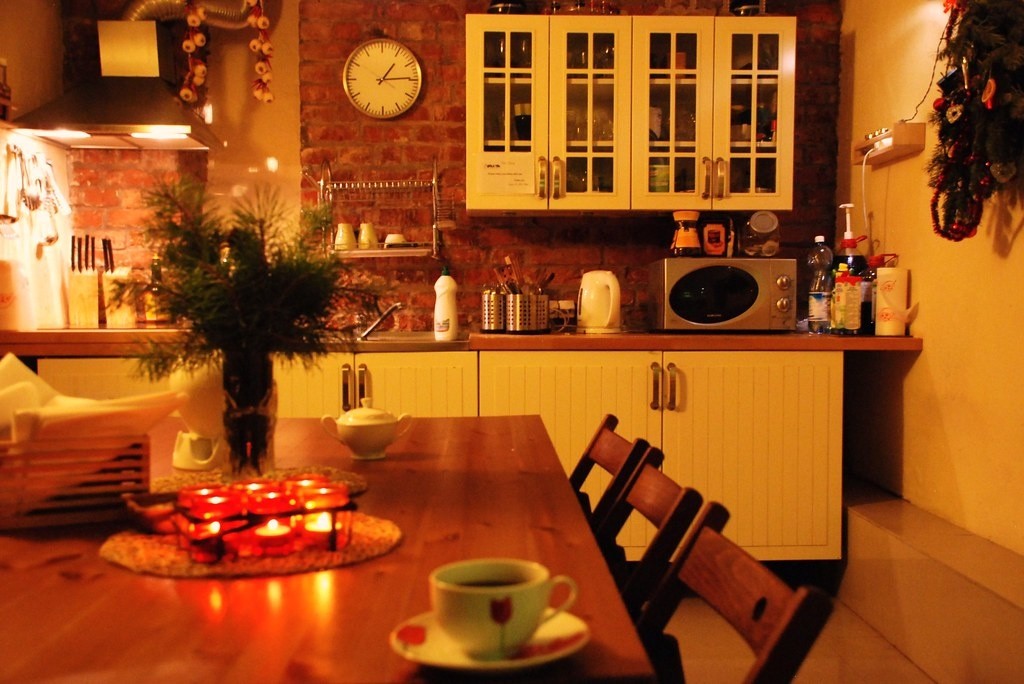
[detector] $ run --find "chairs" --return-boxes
[567,414,833,684]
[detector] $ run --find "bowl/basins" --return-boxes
[383,234,407,249]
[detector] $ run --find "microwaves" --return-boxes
[647,258,797,331]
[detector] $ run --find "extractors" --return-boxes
[12,20,224,151]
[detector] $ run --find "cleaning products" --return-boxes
[433,264,459,342]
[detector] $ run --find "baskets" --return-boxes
[1,428,151,528]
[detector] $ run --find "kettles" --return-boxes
[577,270,620,334]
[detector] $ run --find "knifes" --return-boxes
[71,234,115,274]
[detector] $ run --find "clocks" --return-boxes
[344,39,422,117]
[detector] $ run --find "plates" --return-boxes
[388,606,590,670]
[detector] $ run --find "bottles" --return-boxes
[806,236,884,336]
[143,253,172,323]
[434,266,458,341]
[0,57,11,124]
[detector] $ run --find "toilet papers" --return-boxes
[875,267,908,336]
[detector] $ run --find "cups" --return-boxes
[358,224,378,250]
[428,558,577,662]
[320,397,414,460]
[334,223,356,251]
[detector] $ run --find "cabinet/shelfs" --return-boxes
[40,355,220,401]
[477,348,842,564]
[465,13,797,212]
[272,351,479,419]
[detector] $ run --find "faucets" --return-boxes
[357,301,403,341]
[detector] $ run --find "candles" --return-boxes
[182,472,350,561]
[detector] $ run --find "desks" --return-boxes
[0,415,659,684]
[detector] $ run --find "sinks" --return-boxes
[358,338,436,341]
